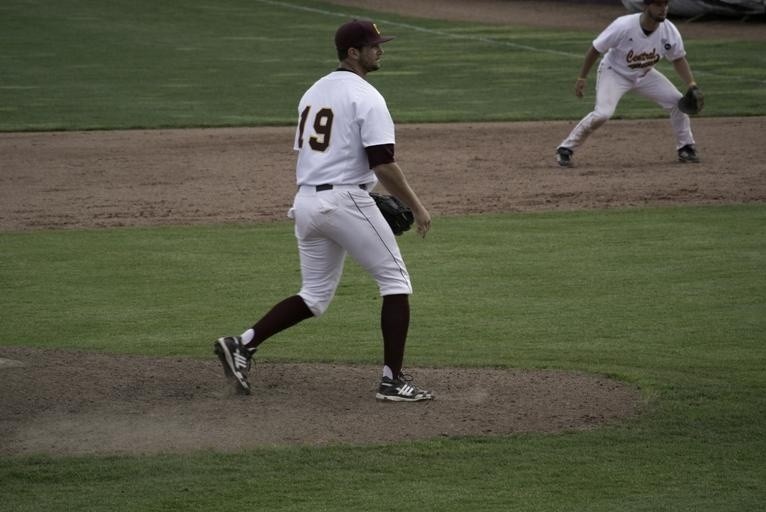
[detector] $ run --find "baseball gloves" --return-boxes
[369,191,414,236]
[678,85,702,113]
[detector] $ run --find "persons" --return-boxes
[550,0,706,167]
[212,14,438,404]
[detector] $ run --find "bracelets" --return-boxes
[577,77,587,82]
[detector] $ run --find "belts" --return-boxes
[315,184,367,190]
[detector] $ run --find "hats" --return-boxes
[335,19,395,47]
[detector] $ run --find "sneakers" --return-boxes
[678,145,700,162]
[214,336,257,394]
[556,147,573,165]
[375,376,433,401]
